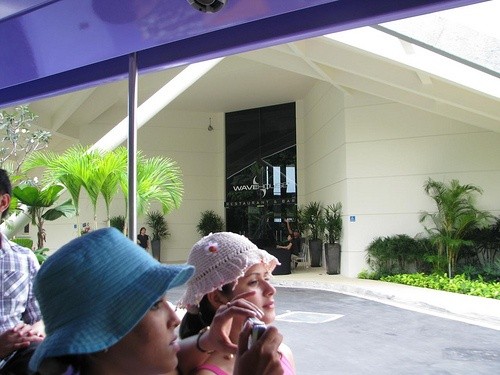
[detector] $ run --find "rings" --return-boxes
[277,350,283,361]
[227,301,231,309]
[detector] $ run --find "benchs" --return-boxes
[291,243,311,268]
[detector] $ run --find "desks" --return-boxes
[259,245,291,275]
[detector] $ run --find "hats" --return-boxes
[28,227,196,374]
[175,231,281,311]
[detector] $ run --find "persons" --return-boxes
[137,227,151,253]
[273,217,301,275]
[27,227,287,375]
[180,232,292,375]
[0,168,48,375]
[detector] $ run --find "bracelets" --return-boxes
[196,326,218,355]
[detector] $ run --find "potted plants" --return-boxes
[143,210,172,262]
[298,200,325,268]
[324,201,342,274]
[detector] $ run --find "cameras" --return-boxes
[248,315,266,348]
